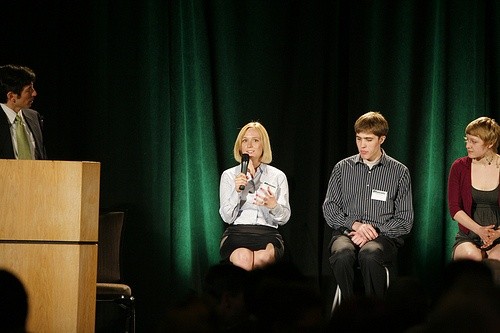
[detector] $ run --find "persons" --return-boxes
[447,116,500,284]
[323,112,414,309]
[0,64,47,160]
[218,121,291,288]
[144,256,500,333]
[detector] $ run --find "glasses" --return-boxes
[464,136,485,145]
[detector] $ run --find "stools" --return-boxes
[327,264,390,314]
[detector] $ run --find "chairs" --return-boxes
[95,210,137,333]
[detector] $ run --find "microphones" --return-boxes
[239,154,249,190]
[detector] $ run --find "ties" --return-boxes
[14,115,33,160]
